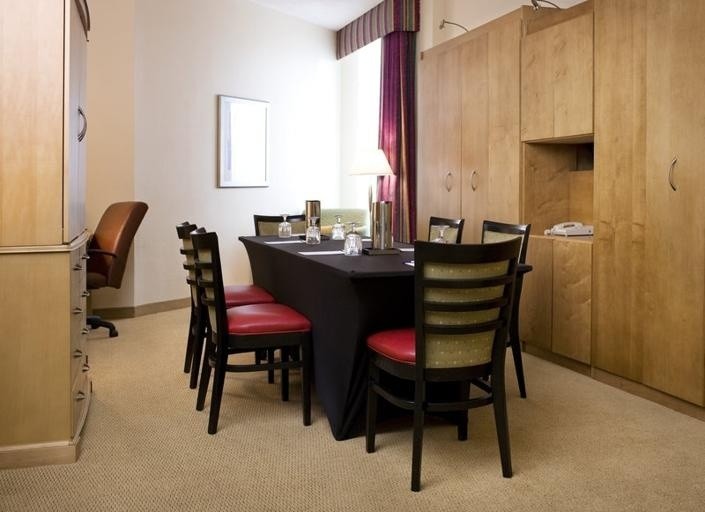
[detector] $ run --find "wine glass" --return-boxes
[430,224,450,243]
[278,214,363,256]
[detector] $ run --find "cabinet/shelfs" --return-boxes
[419,4,521,351]
[521,1,594,380]
[1,242,90,472]
[0,0,90,246]
[593,0,705,423]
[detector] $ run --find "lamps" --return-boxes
[348,149,394,239]
[531,0,560,11]
[440,19,468,33]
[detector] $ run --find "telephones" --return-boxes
[551,222,593,236]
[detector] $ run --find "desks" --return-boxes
[240,236,532,440]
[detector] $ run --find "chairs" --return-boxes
[254,214,307,236]
[86,201,149,338]
[191,227,313,434]
[366,236,523,492]
[430,216,464,244]
[177,221,275,388]
[481,220,532,398]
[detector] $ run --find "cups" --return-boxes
[371,201,392,249]
[305,200,321,235]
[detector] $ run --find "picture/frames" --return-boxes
[218,94,271,188]
